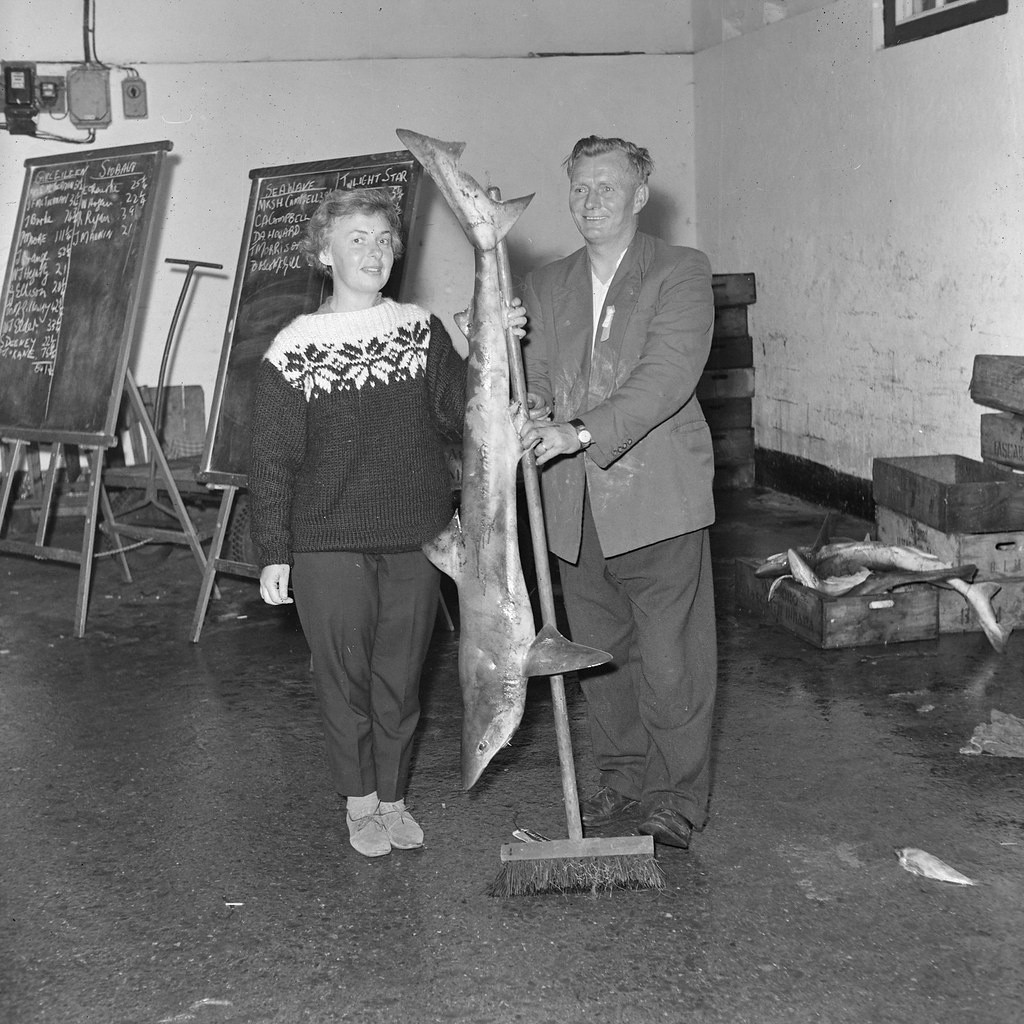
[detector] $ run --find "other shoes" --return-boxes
[346,811,392,857]
[380,803,424,850]
[637,808,691,847]
[577,786,638,830]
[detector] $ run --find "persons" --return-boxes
[514,135,716,848]
[248,190,527,858]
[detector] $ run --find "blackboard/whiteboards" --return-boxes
[196,148,423,491]
[0,138,175,449]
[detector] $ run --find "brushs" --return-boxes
[486,183,662,897]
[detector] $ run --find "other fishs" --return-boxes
[754,510,1013,655]
[397,126,612,794]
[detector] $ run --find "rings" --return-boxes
[542,442,547,451]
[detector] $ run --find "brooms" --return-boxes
[483,185,665,900]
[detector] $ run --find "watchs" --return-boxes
[567,418,592,449]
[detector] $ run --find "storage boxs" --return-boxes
[694,273,756,490]
[734,356,1024,650]
